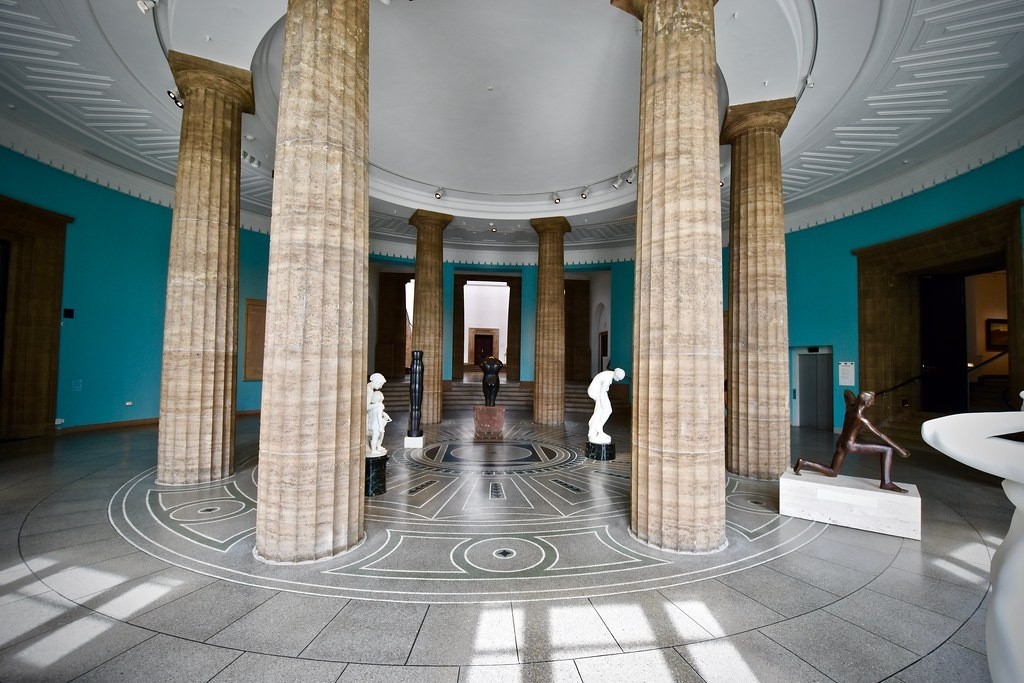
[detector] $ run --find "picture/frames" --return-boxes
[985,318,1008,352]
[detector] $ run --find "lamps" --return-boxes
[580,187,591,198]
[807,75,813,88]
[435,188,444,199]
[167,88,180,100]
[551,192,560,204]
[487,224,497,232]
[613,176,623,189]
[720,178,725,188]
[175,98,184,109]
[136,0,158,14]
[626,171,637,184]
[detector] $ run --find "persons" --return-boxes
[367,373,392,453]
[479,356,503,407]
[921,392,1023,682]
[588,367,625,440]
[794,389,910,492]
